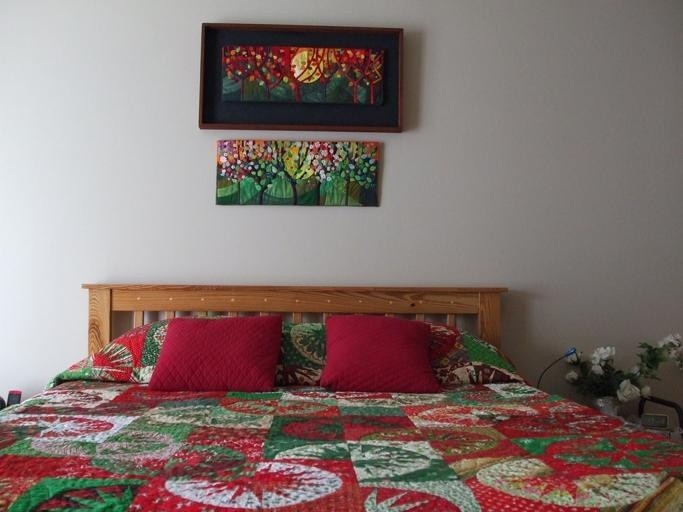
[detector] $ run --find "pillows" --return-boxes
[319,313,444,392]
[143,313,284,393]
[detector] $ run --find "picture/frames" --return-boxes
[198,25,404,131]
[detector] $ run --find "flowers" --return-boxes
[563,330,683,407]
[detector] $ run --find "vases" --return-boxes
[593,396,618,418]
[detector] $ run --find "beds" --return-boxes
[0,282,683,512]
